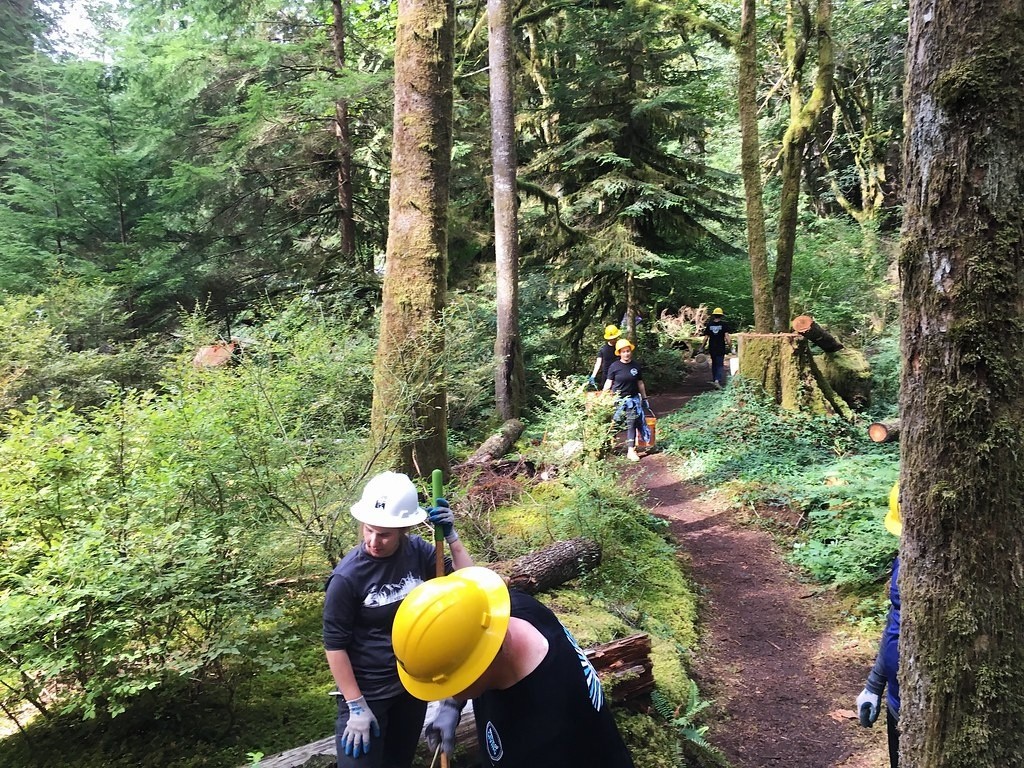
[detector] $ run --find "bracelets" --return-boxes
[642,397,647,400]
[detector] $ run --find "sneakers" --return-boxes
[627,446,640,461]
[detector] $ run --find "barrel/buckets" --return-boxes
[635,417,656,447]
[585,390,613,408]
[695,353,709,371]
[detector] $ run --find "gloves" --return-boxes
[589,377,595,385]
[340,695,380,759]
[856,688,881,728]
[642,398,649,408]
[428,696,467,760]
[730,347,736,354]
[425,497,458,543]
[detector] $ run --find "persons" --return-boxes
[322,471,473,767]
[857,482,902,768]
[391,566,633,767]
[604,338,650,461]
[699,307,734,391]
[589,325,622,387]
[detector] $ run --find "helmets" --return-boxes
[712,308,723,315]
[350,472,428,528]
[391,566,511,701]
[615,339,635,356]
[604,325,621,339]
[885,481,902,536]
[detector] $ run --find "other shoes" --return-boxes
[714,382,721,390]
